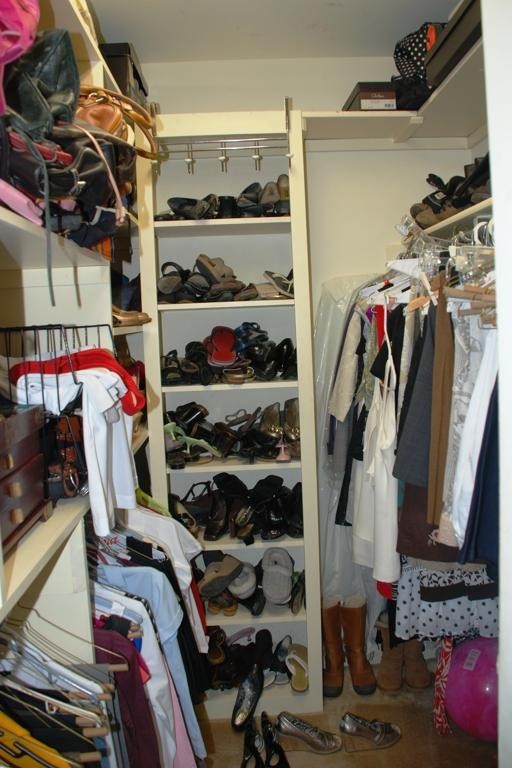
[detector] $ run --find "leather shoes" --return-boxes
[231,663,265,731]
[112,303,152,327]
[240,710,402,768]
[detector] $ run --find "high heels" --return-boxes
[163,396,305,546]
[154,173,290,221]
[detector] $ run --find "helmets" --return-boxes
[442,636,499,743]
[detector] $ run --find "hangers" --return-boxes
[368,218,495,332]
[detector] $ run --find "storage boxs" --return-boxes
[100,39,151,108]
[422,3,484,92]
[343,77,400,117]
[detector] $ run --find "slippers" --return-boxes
[200,625,310,692]
[157,252,294,305]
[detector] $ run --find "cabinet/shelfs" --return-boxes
[0,0,122,632]
[131,97,329,727]
[401,30,498,243]
[102,55,174,522]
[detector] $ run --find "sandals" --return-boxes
[161,322,297,386]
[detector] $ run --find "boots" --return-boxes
[321,598,432,698]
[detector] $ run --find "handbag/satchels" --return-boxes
[0,0,157,246]
[389,21,450,110]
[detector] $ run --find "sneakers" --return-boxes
[190,547,306,616]
[410,151,491,232]
